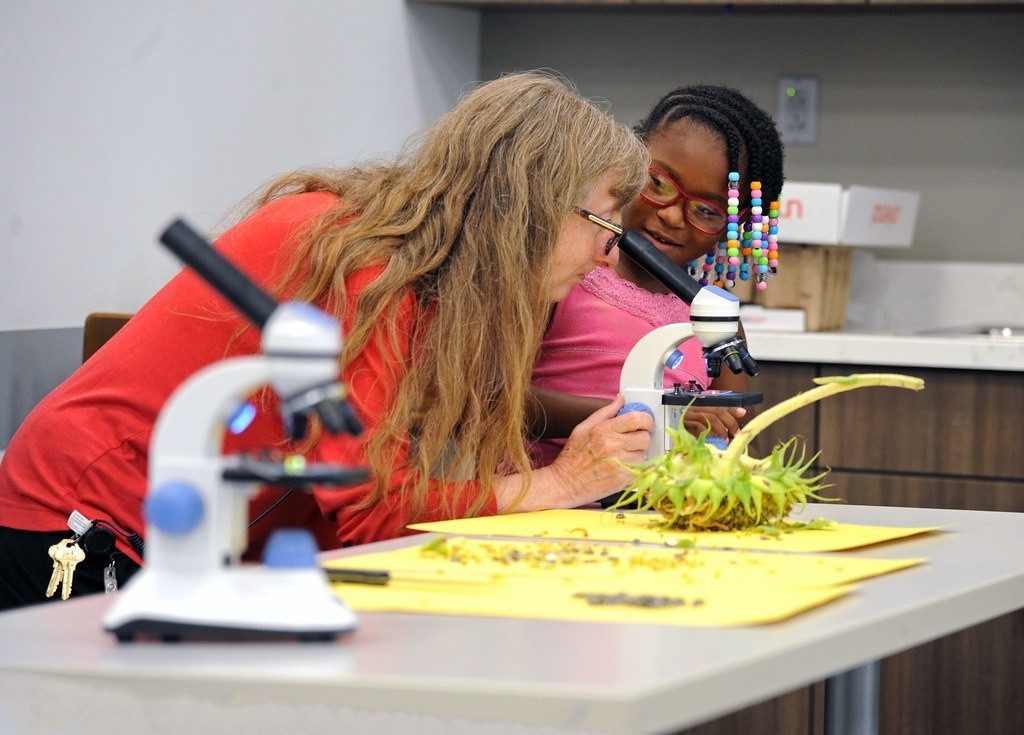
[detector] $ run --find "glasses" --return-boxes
[641,168,749,234]
[571,204,631,255]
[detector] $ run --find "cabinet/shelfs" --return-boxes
[712,363,1024,735]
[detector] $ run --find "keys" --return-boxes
[46,539,67,597]
[54,540,85,600]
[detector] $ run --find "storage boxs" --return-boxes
[696,242,854,329]
[761,182,921,251]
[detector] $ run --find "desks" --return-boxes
[0,499,1024,735]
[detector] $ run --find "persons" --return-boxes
[0,68,655,612]
[497,83,785,480]
